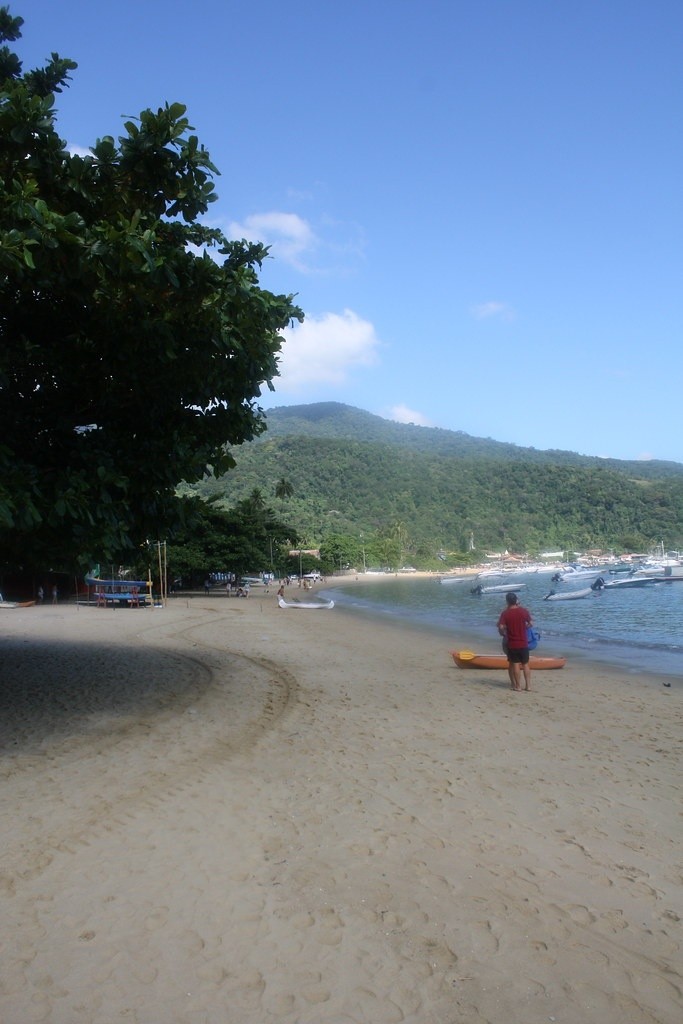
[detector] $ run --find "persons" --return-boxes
[244,581,250,598]
[278,585,284,596]
[499,597,523,690]
[226,583,231,596]
[204,579,209,596]
[497,593,534,692]
[52,583,58,605]
[261,572,327,595]
[237,589,243,596]
[37,585,43,604]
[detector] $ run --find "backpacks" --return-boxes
[527,626,541,651]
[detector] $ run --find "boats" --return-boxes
[0,593,36,608]
[436,579,463,584]
[604,577,655,588]
[609,567,637,574]
[551,566,607,582]
[453,650,568,670]
[470,584,526,594]
[543,577,605,602]
[277,595,334,609]
